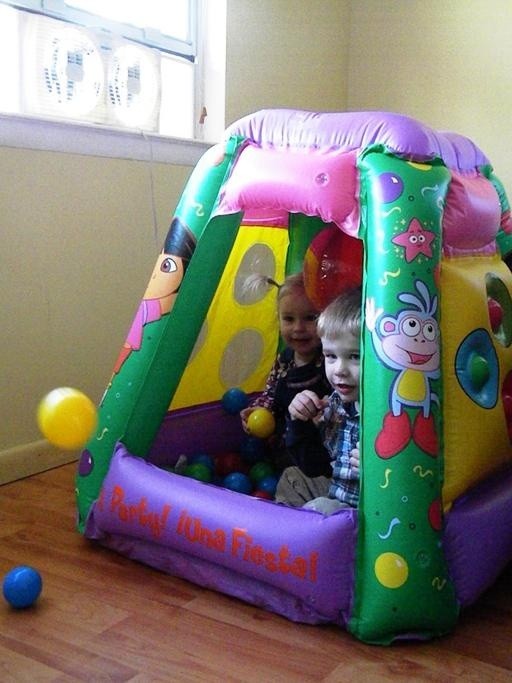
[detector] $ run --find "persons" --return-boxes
[239,271,333,478]
[274,287,361,517]
